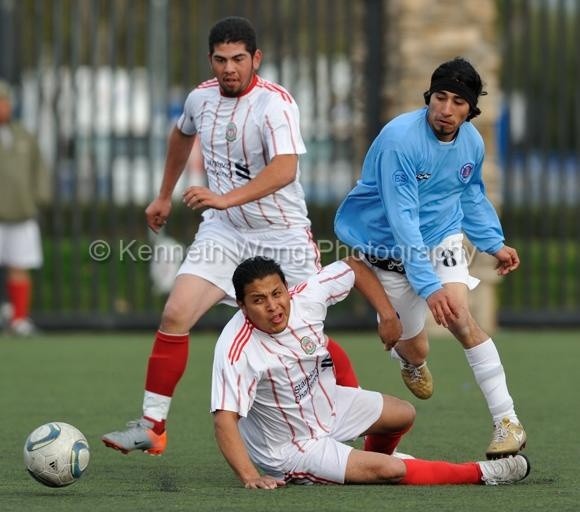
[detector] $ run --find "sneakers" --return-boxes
[389,345,436,402]
[485,416,529,462]
[475,452,532,487]
[391,448,417,462]
[8,317,38,338]
[100,415,168,457]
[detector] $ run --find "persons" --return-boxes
[208,255,531,492]
[102,15,324,457]
[333,56,529,458]
[1,78,44,339]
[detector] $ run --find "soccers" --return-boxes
[24,422,89,487]
